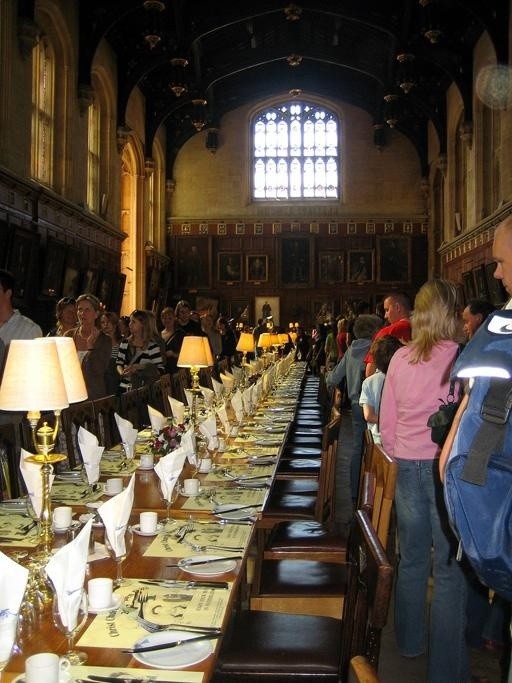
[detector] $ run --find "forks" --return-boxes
[134,617,220,633]
[190,541,244,553]
[138,593,151,618]
[173,515,194,545]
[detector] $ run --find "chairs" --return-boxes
[212,361,400,683]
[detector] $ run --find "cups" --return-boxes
[139,511,159,532]
[184,478,200,494]
[199,458,211,469]
[53,506,73,530]
[141,453,154,467]
[24,651,71,682]
[86,576,113,606]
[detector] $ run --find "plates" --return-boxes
[12,671,71,682]
[133,630,213,669]
[180,491,202,497]
[86,592,126,613]
[133,523,165,536]
[138,463,156,470]
[1,434,153,509]
[79,512,103,527]
[204,362,308,504]
[213,503,257,519]
[178,555,238,577]
[198,466,216,474]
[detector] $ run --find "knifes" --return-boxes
[74,674,189,683]
[208,503,263,516]
[166,554,243,569]
[138,578,228,591]
[122,633,226,655]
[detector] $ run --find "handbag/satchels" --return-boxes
[442,309,512,604]
[427,403,462,445]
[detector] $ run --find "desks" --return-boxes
[0,360,306,682]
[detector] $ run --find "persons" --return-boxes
[316,216,509,683]
[1,272,240,501]
[231,318,324,377]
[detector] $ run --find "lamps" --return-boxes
[372,0,443,155]
[144,0,220,156]
[176,331,297,419]
[284,0,304,98]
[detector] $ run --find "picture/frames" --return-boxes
[456,261,507,313]
[246,254,269,283]
[318,251,345,283]
[193,292,283,327]
[274,231,317,291]
[310,291,388,326]
[347,249,375,283]
[376,234,412,286]
[217,251,243,283]
[175,235,212,290]
[1,221,125,317]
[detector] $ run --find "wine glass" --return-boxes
[9,549,51,641]
[158,476,179,524]
[51,590,90,664]
[103,523,134,587]
